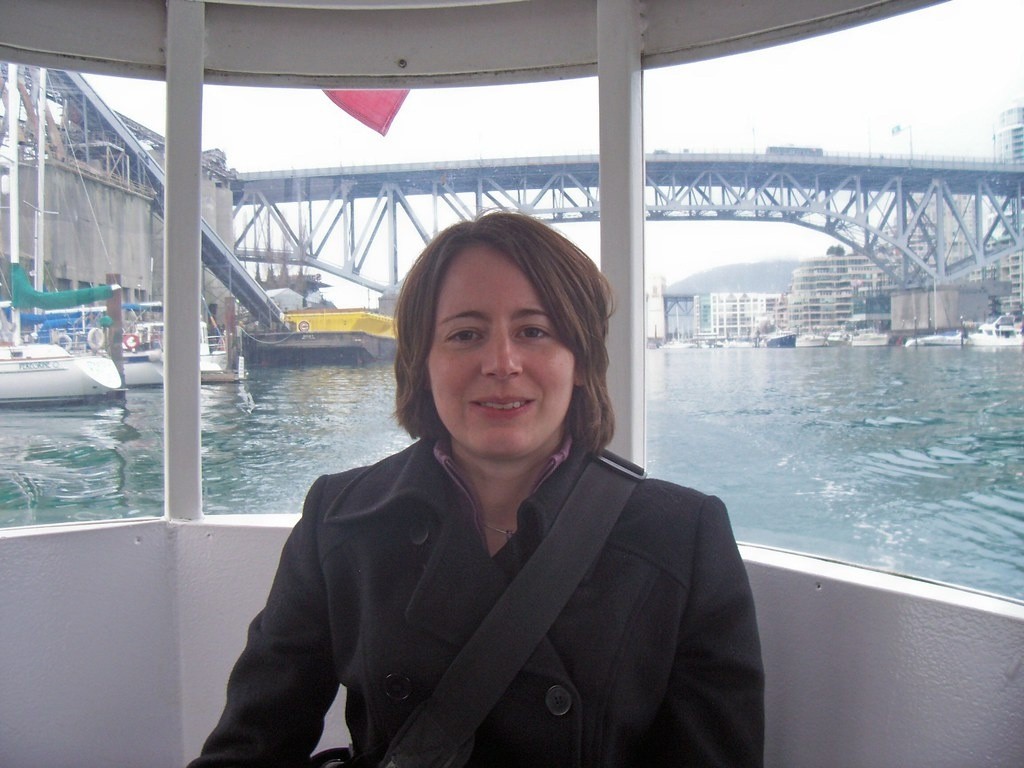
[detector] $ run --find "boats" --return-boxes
[827,332,849,346]
[904,331,965,348]
[762,332,795,348]
[795,335,826,347]
[852,333,890,347]
[968,313,1024,346]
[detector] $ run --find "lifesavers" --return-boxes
[123,333,141,350]
[53,333,72,354]
[87,327,106,351]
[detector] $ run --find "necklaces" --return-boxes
[472,521,523,543]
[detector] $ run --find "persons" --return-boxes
[187,211,766,768]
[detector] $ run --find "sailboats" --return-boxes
[0,60,236,406]
